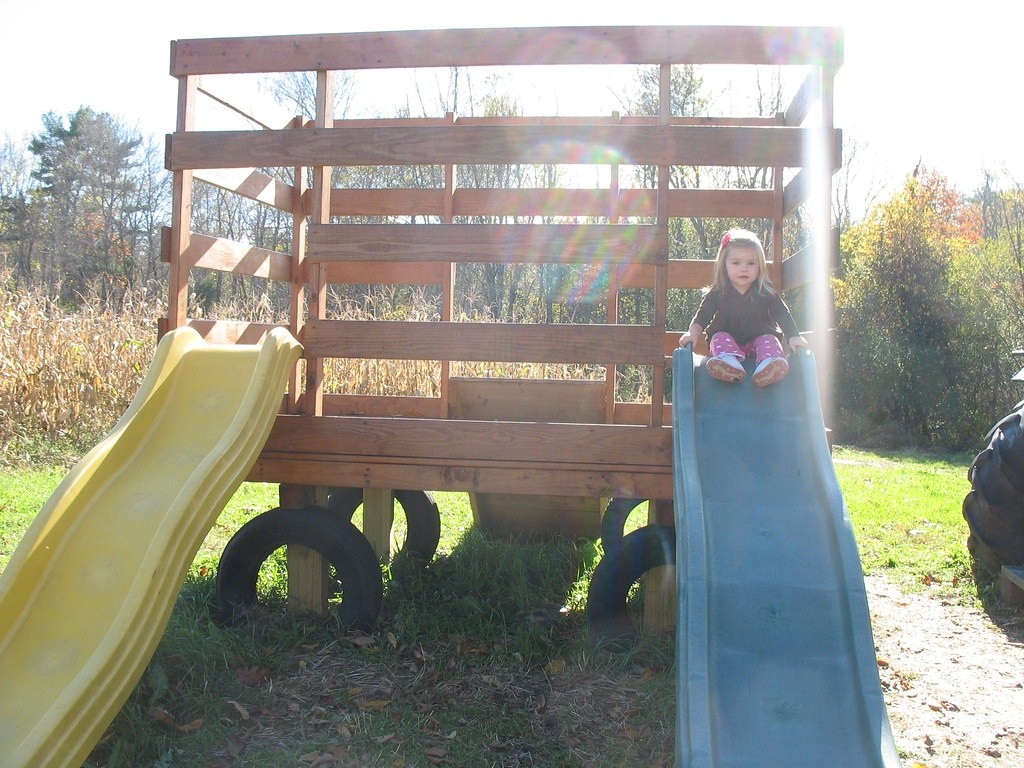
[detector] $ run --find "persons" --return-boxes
[676,225,810,387]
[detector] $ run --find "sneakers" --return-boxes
[752,357,790,388]
[706,357,746,382]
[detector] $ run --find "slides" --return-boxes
[0,325,303,767]
[672,344,907,768]
